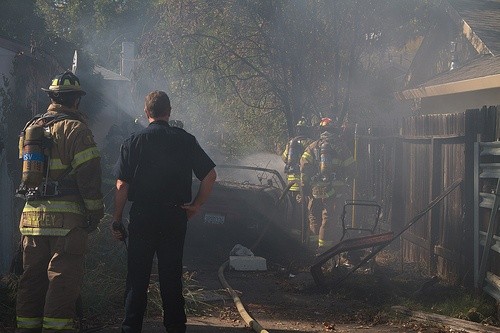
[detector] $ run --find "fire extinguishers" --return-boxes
[321,143,331,182]
[19,126,46,199]
[288,139,298,172]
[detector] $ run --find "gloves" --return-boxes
[87,218,100,233]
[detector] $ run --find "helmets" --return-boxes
[41,69,86,97]
[319,116,337,127]
[296,117,312,127]
[104,124,126,139]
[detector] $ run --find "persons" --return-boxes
[12,69,106,333]
[111,89,218,333]
[281,117,317,193]
[100,123,125,206]
[301,116,355,256]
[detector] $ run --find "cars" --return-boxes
[191,165,296,242]
